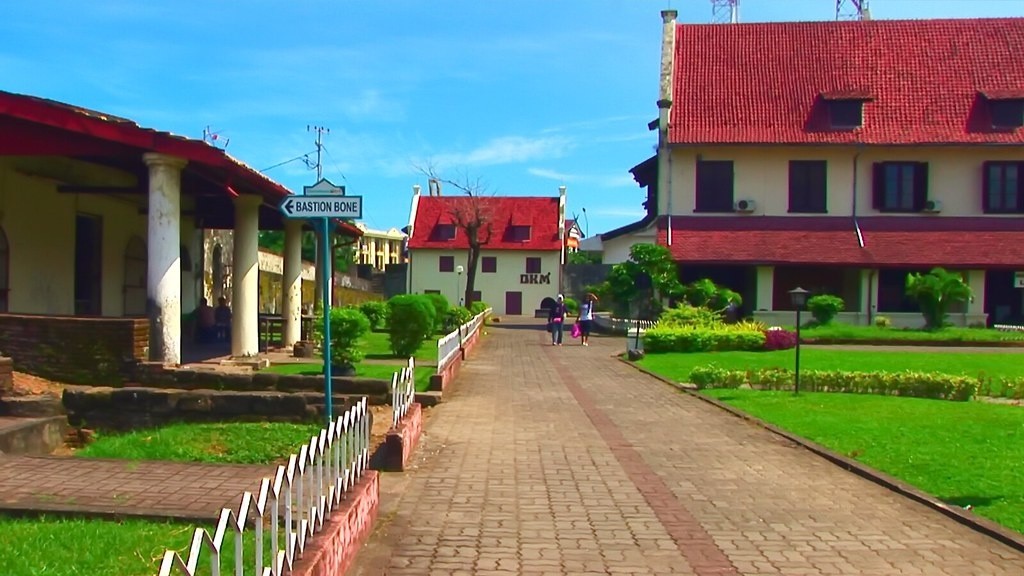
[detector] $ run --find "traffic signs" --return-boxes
[280,195,363,218]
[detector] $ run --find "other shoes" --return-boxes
[558,344,562,346]
[582,341,588,346]
[552,342,556,345]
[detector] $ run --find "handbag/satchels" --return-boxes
[553,317,562,322]
[591,315,596,321]
[547,322,552,332]
[571,323,579,338]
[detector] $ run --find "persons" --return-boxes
[214,296,232,340]
[575,292,599,346]
[189,297,217,344]
[548,293,571,346]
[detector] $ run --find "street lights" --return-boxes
[456,265,464,307]
[787,287,811,394]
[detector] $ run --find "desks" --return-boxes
[260,315,317,354]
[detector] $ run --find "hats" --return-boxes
[557,294,563,301]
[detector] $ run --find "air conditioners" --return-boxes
[925,198,942,213]
[737,199,756,211]
